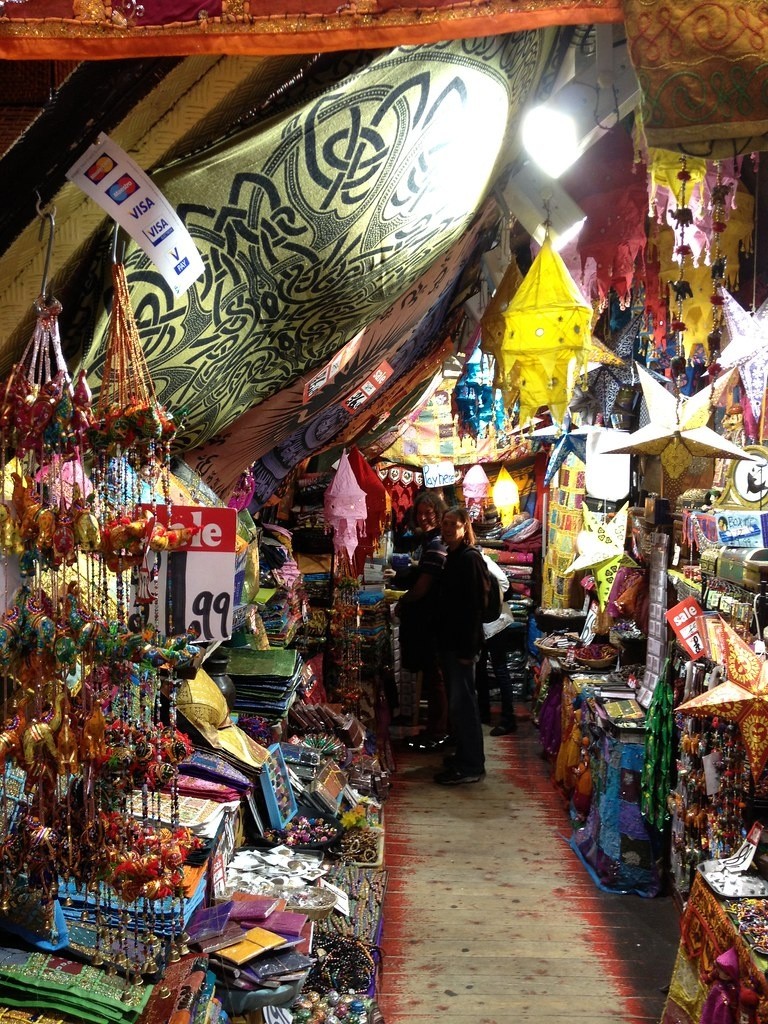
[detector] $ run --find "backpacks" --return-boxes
[464,548,503,623]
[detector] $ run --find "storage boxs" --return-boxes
[258,743,298,830]
[700,545,768,592]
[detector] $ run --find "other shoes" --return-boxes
[489,722,518,736]
[421,727,444,741]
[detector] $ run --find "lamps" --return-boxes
[502,165,588,253]
[493,466,521,527]
[520,22,642,180]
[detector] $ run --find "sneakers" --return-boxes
[434,762,481,785]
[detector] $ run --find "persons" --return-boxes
[477,543,518,736]
[383,490,449,753]
[433,508,491,786]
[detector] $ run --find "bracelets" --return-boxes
[311,935,378,996]
